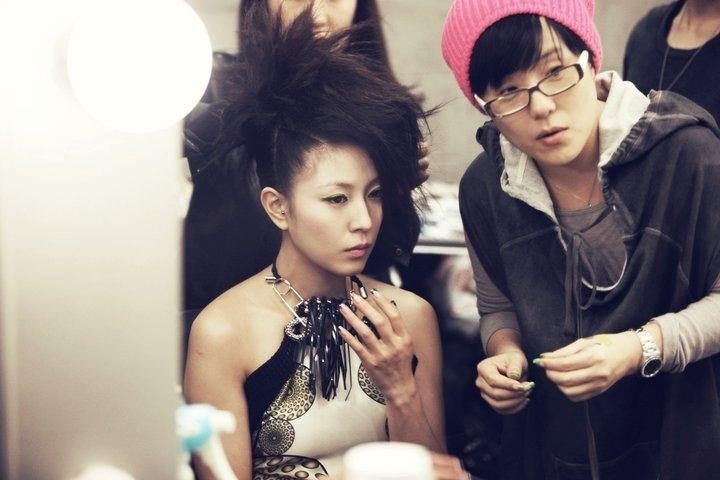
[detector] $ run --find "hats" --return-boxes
[439,0,606,117]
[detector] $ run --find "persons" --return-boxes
[621,1,719,132]
[441,1,720,480]
[184,1,430,311]
[183,1,473,480]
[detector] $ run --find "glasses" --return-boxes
[471,48,592,121]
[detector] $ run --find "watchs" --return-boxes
[630,327,664,381]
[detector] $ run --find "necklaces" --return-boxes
[269,261,365,402]
[544,157,599,207]
[658,4,718,90]
